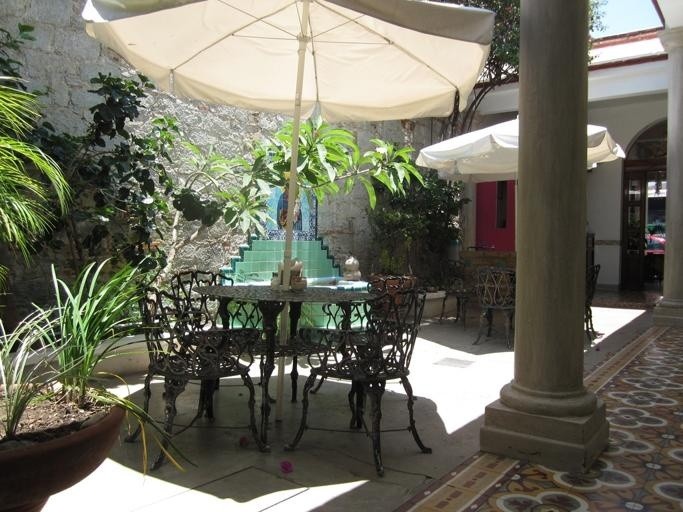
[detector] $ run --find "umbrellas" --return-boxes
[82,2,495,291]
[412,115,625,185]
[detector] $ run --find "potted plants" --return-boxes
[0,249,197,512]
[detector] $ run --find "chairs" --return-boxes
[437,246,600,350]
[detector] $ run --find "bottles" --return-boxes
[269,272,280,291]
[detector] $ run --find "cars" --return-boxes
[643,223,667,256]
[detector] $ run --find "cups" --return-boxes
[289,276,307,293]
